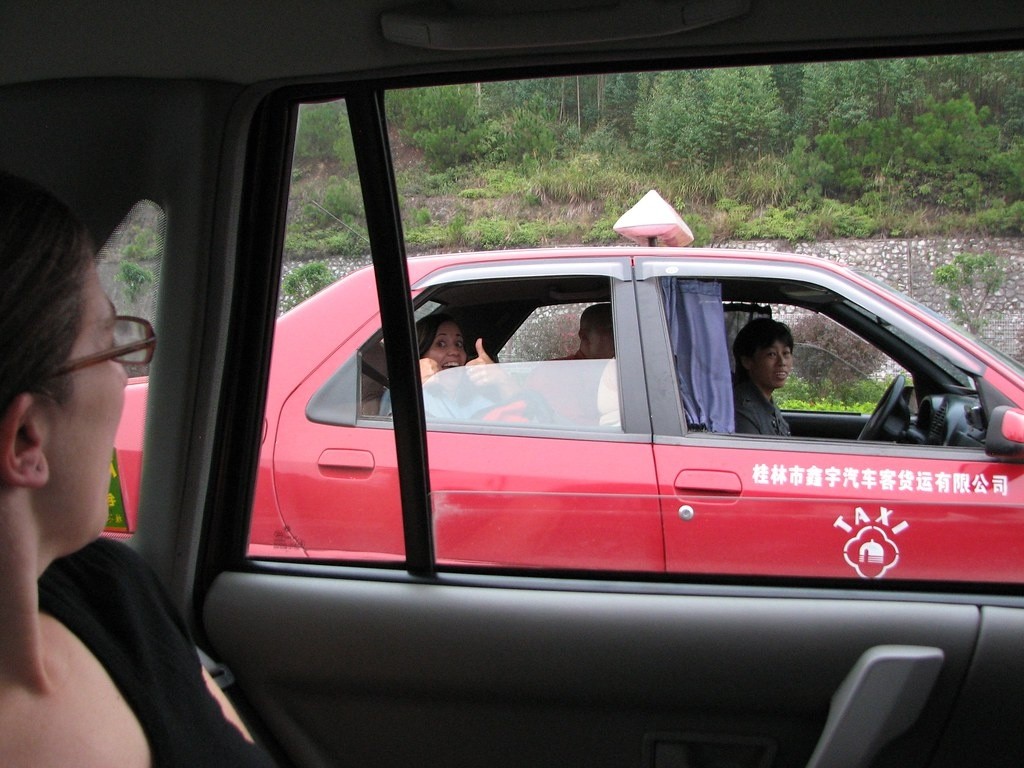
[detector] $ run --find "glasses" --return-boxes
[36,315,157,385]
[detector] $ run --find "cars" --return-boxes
[100,187,1023,585]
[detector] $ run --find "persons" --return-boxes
[0,164,293,768]
[733,317,794,438]
[378,312,554,426]
[528,303,615,425]
